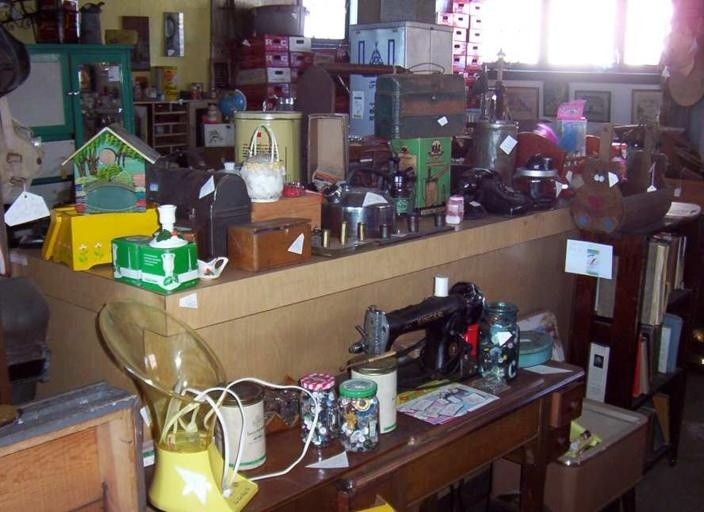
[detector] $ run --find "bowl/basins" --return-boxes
[516,330,555,369]
[250,6,309,37]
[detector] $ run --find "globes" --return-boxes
[219,88,247,124]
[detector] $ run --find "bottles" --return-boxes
[300,353,398,454]
[481,301,518,383]
[513,155,562,212]
[388,168,415,217]
[215,380,266,472]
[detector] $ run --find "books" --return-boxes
[588,229,688,463]
